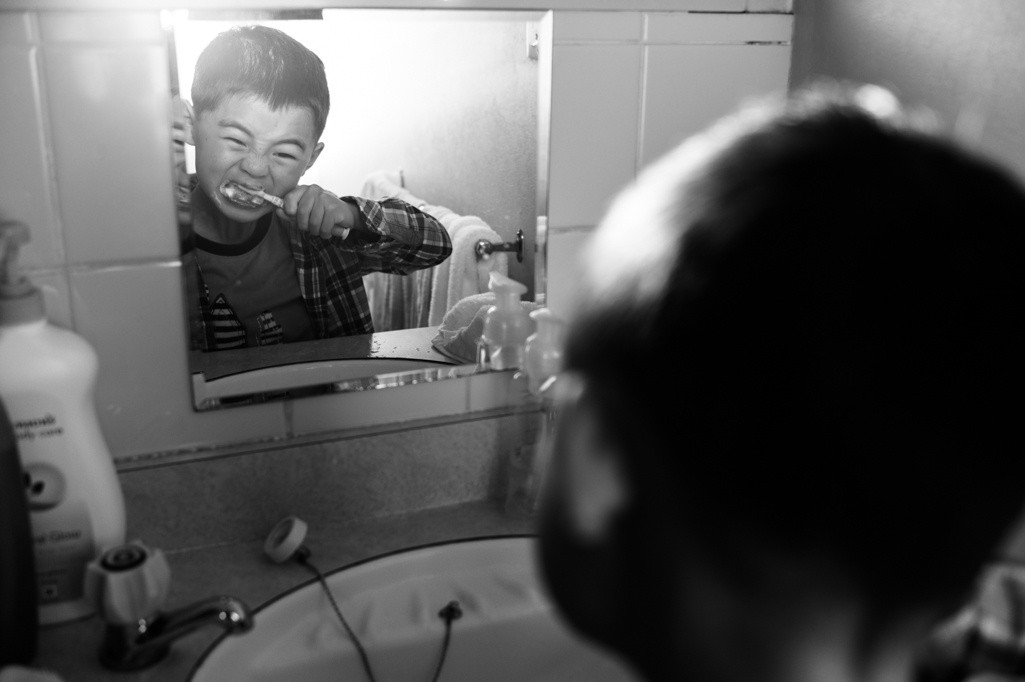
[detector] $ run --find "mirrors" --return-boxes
[158,3,555,414]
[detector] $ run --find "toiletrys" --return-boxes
[0,220,135,627]
[477,266,528,372]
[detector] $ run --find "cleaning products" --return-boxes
[502,309,571,520]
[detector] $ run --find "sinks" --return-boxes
[191,355,454,404]
[185,533,648,680]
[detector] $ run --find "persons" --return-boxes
[531,88,1024,682]
[181,26,455,353]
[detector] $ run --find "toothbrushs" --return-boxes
[229,182,352,242]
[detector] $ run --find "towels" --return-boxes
[433,291,541,363]
[343,168,511,329]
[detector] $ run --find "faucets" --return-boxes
[82,537,254,671]
